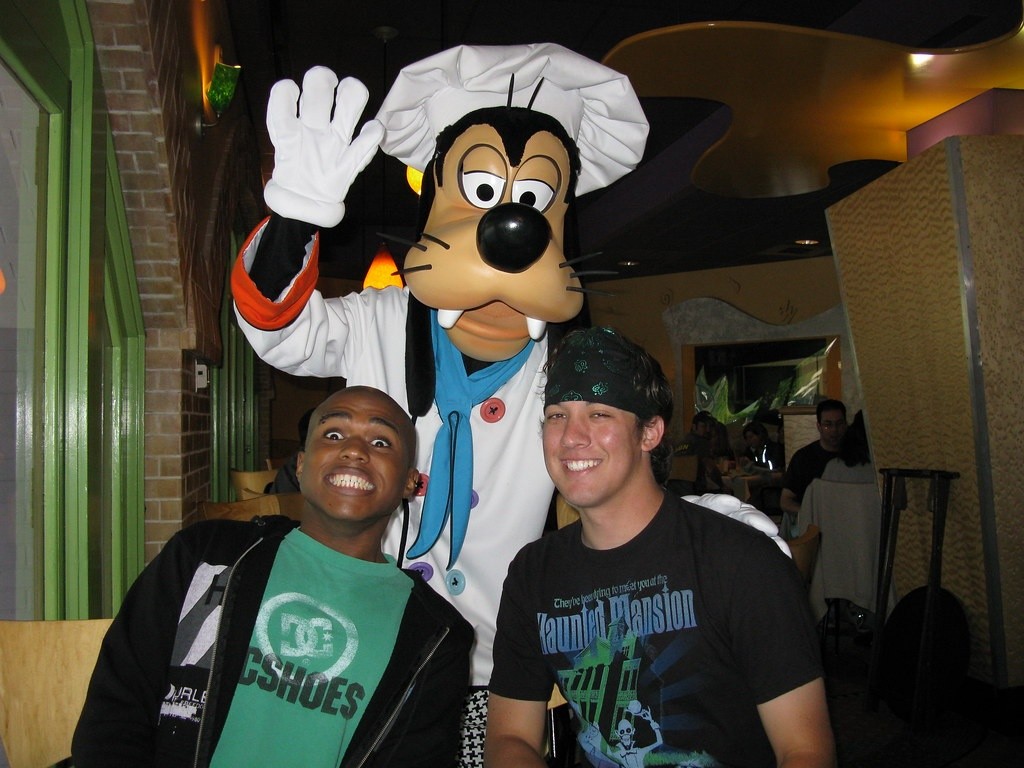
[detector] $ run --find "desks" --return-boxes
[705,473,784,502]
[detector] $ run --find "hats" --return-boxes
[693,411,718,425]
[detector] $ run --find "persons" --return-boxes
[674,398,875,514]
[269,407,315,494]
[484,327,838,768]
[46,385,474,768]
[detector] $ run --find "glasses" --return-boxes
[819,423,845,432]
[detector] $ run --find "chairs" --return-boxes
[0,618,115,768]
[198,491,304,522]
[667,455,704,495]
[231,457,291,501]
[798,477,882,662]
[788,525,821,595]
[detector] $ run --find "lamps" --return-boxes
[194,61,241,141]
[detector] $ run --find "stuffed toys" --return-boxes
[232,41,792,768]
